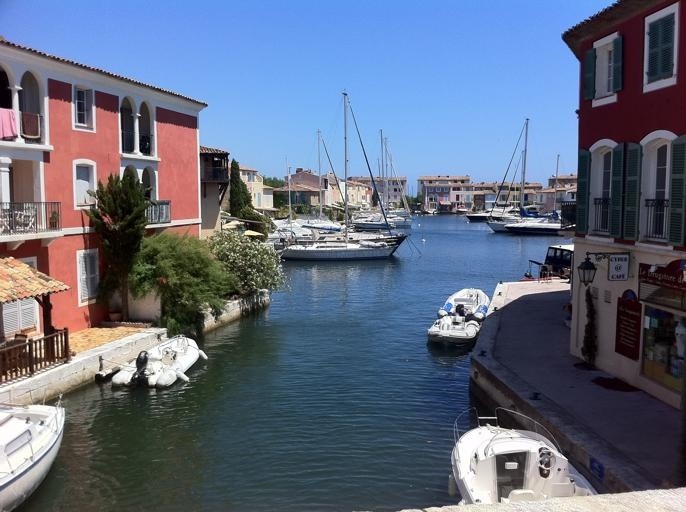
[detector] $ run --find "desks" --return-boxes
[0,338,26,349]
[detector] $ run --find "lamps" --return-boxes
[649,264,668,272]
[578,252,622,287]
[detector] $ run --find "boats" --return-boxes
[0,386,65,512]
[112,333,200,389]
[522,245,573,281]
[428,288,490,343]
[449,406,598,504]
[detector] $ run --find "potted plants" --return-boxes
[49,211,58,229]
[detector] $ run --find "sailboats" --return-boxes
[259,89,413,259]
[466,117,562,234]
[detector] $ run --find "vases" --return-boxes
[108,313,122,321]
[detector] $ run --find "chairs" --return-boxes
[538,265,552,283]
[0,203,37,235]
[15,335,27,338]
[501,483,587,504]
